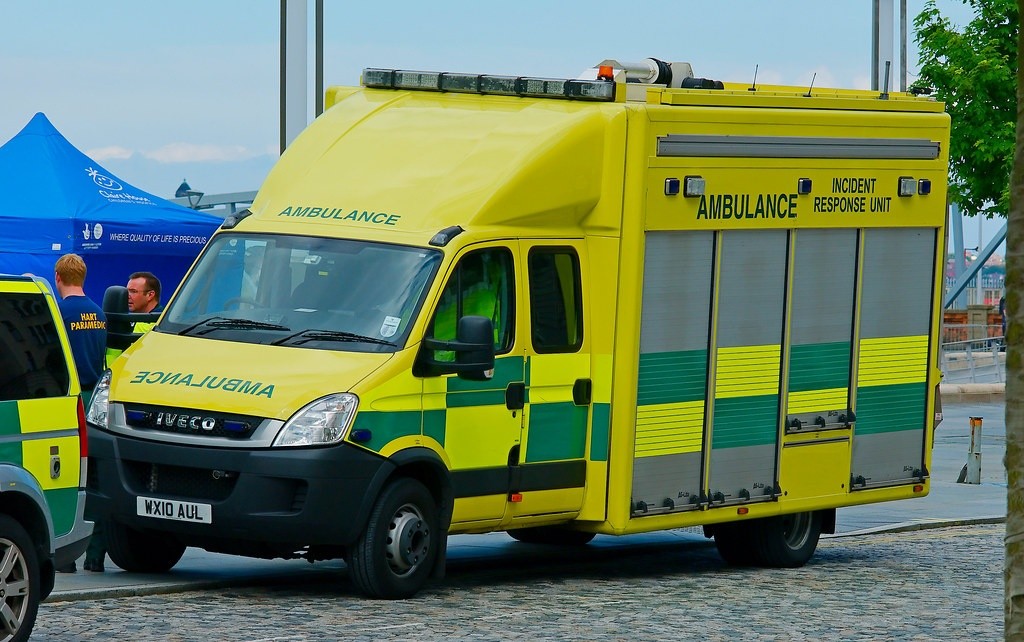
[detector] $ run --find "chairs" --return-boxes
[510,263,575,348]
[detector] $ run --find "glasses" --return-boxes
[127,289,153,294]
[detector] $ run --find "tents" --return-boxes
[0,113,245,323]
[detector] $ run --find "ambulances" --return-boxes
[83,55,952,602]
[0,272,95,642]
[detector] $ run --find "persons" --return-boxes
[103,272,164,372]
[54,253,107,573]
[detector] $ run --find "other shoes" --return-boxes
[61,563,76,573]
[84,557,104,572]
